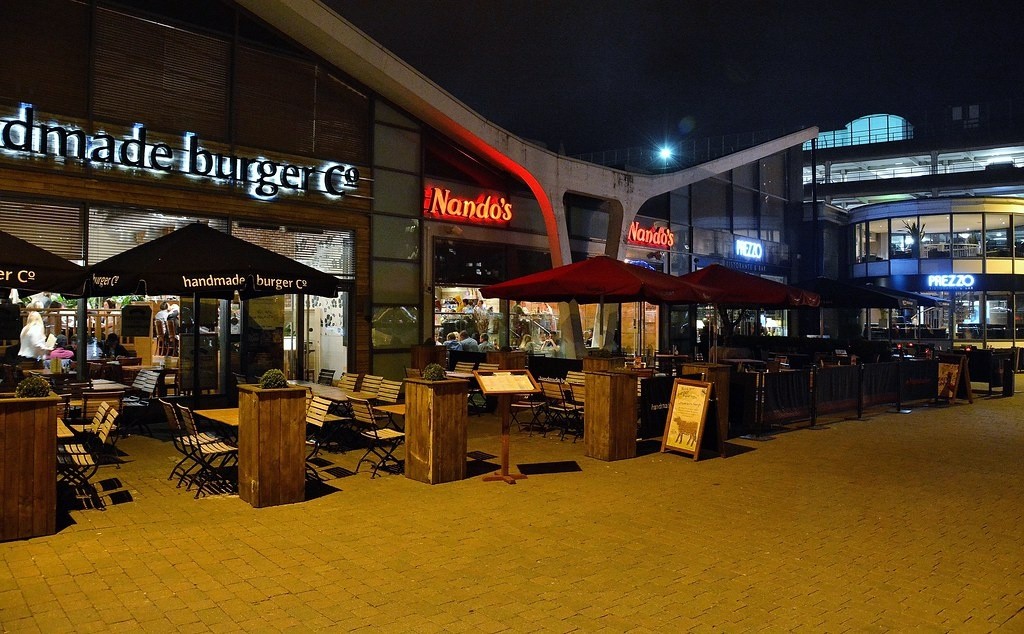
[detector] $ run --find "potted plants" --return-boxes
[611,350,627,369]
[410,338,448,370]
[236,368,312,508]
[0,377,63,541]
[402,363,471,483]
[582,349,617,369]
[486,346,527,370]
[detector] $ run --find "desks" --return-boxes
[56,417,75,439]
[532,348,556,358]
[372,403,411,433]
[193,407,239,472]
[285,379,339,388]
[22,357,165,392]
[307,388,380,418]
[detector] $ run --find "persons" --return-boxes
[156,301,180,357]
[17,311,56,370]
[433,299,595,357]
[480,253,952,359]
[699,319,714,360]
[33,298,129,360]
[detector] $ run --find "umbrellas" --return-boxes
[84,221,353,418]
[0,227,84,403]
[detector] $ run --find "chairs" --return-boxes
[177,403,239,500]
[57,407,119,501]
[404,348,881,444]
[70,391,124,469]
[56,393,72,426]
[158,397,236,488]
[118,350,160,431]
[306,369,405,479]
[56,401,109,486]
